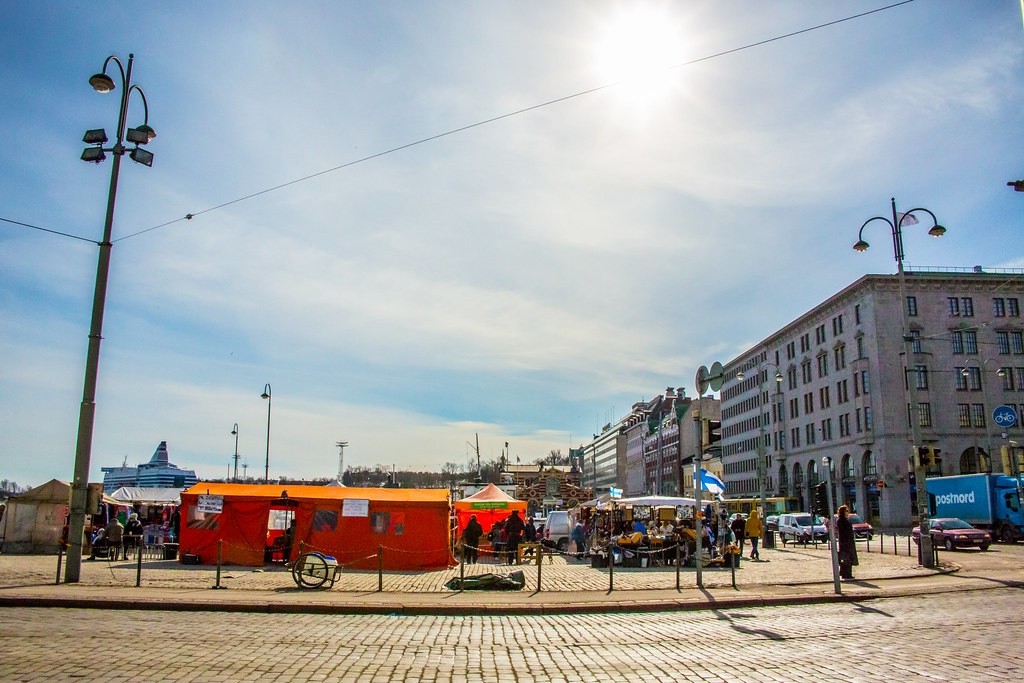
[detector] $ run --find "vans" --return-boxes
[779,513,828,545]
[540,511,571,556]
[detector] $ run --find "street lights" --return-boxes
[735,354,783,519]
[853,198,946,570]
[64,53,156,585]
[231,423,239,483]
[260,383,272,485]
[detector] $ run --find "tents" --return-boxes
[110,486,190,507]
[452,483,528,557]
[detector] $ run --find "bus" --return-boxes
[719,497,800,522]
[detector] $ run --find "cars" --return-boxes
[766,515,779,530]
[729,513,749,523]
[911,517,992,551]
[824,514,874,540]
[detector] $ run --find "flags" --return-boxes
[693,464,726,494]
[610,487,623,499]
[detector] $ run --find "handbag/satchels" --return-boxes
[837,550,851,566]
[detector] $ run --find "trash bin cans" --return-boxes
[762,530,774,548]
[163,543,179,560]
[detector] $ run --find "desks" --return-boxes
[518,544,540,564]
[124,536,144,560]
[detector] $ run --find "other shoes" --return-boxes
[842,574,855,579]
[756,552,759,559]
[750,554,755,559]
[494,557,499,561]
[87,557,95,560]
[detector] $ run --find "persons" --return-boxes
[523,517,537,560]
[836,505,859,578]
[745,509,764,559]
[627,519,674,534]
[501,517,509,541]
[464,514,483,564]
[505,508,525,568]
[105,518,123,561]
[731,513,745,558]
[571,519,585,559]
[86,528,111,560]
[122,513,143,560]
[282,519,296,562]
[490,521,503,561]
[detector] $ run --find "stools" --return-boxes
[107,544,166,561]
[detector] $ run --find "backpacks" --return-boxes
[488,528,497,541]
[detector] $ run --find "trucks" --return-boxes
[924,473,1024,545]
